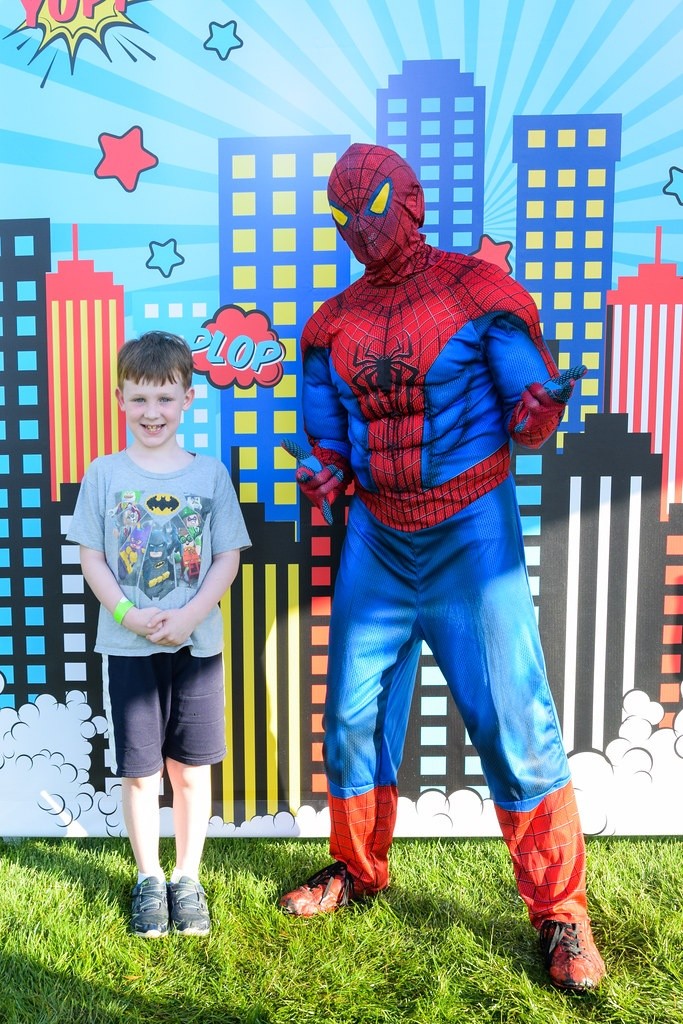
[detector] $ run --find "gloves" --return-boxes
[514,365,588,434]
[280,438,345,524]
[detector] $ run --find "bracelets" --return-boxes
[114,597,134,625]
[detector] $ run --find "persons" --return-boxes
[64,332,252,938]
[281,143,607,989]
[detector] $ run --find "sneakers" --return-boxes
[130,875,169,938]
[279,859,384,917]
[166,874,210,935]
[539,919,606,990]
